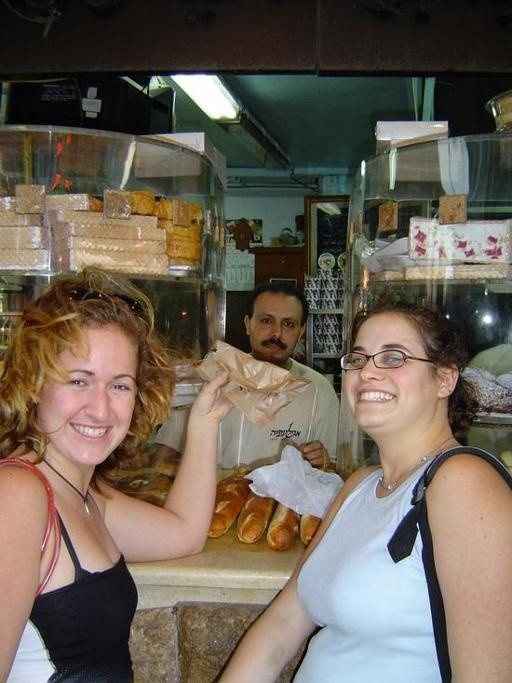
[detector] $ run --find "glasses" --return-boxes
[64,286,150,325]
[338,349,437,370]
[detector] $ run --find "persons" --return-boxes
[176,278,339,471]
[213,292,511,683]
[0,260,237,683]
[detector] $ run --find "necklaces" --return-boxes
[27,444,93,517]
[370,453,440,491]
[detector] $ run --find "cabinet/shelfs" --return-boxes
[346,130,512,423]
[0,121,229,423]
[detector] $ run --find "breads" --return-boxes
[113,450,321,552]
[462,367,512,416]
